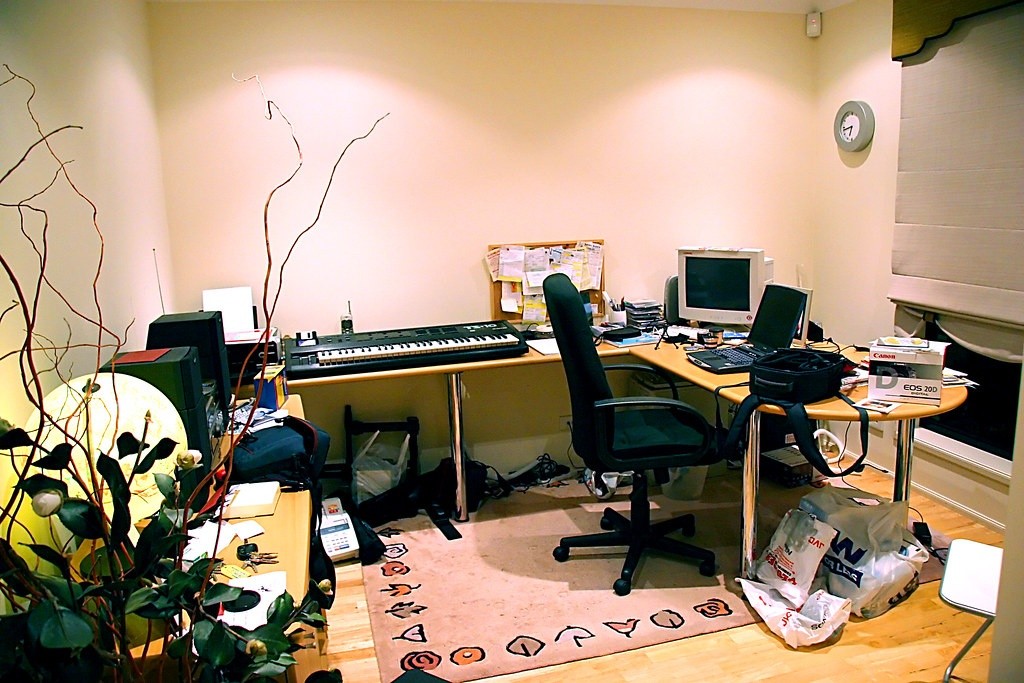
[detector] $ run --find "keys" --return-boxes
[237,538,280,572]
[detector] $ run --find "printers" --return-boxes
[198,307,281,390]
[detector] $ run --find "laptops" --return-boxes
[686,285,807,374]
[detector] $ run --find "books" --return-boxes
[605,333,660,348]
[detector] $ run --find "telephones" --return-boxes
[340,300,354,334]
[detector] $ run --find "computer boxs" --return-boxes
[100,312,232,480]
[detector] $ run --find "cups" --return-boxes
[611,310,627,327]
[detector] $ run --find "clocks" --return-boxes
[834,101,874,151]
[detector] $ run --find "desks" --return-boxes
[232,337,967,582]
[125,393,328,683]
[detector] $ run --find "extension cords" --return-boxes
[507,459,542,480]
[539,469,579,486]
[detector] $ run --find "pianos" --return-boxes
[284,319,533,381]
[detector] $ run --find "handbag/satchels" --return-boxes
[734,484,930,651]
[351,429,412,507]
[224,414,331,488]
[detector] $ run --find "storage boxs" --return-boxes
[220,481,281,519]
[760,445,829,488]
[867,336,946,406]
[254,364,288,412]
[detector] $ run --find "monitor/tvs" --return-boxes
[678,248,775,333]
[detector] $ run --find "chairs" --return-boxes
[939,537,1003,683]
[542,273,720,597]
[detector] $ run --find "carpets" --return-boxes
[360,474,783,683]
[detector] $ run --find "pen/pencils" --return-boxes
[610,296,625,311]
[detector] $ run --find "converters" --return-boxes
[912,521,932,545]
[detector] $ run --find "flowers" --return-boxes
[0,378,343,683]
